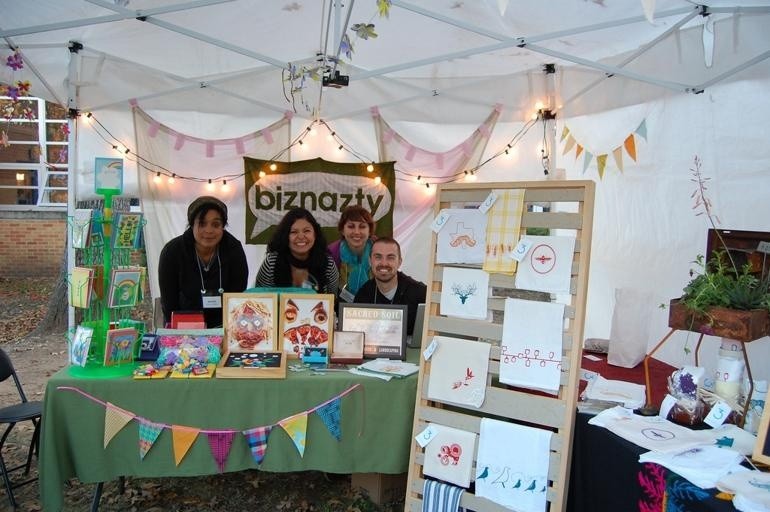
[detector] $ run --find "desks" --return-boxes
[566,347,770,512]
[39,350,420,511]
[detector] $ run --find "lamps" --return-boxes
[16,173,25,194]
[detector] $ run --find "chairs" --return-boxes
[0,349,44,512]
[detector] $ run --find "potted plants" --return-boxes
[657,151,770,356]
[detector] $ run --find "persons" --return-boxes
[327,204,378,294]
[254,207,339,300]
[158,195,249,328]
[353,236,428,336]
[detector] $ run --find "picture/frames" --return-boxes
[279,292,334,358]
[339,302,408,363]
[751,386,769,469]
[222,292,279,353]
[331,331,365,364]
[215,349,287,379]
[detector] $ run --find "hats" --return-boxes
[187,196,228,223]
